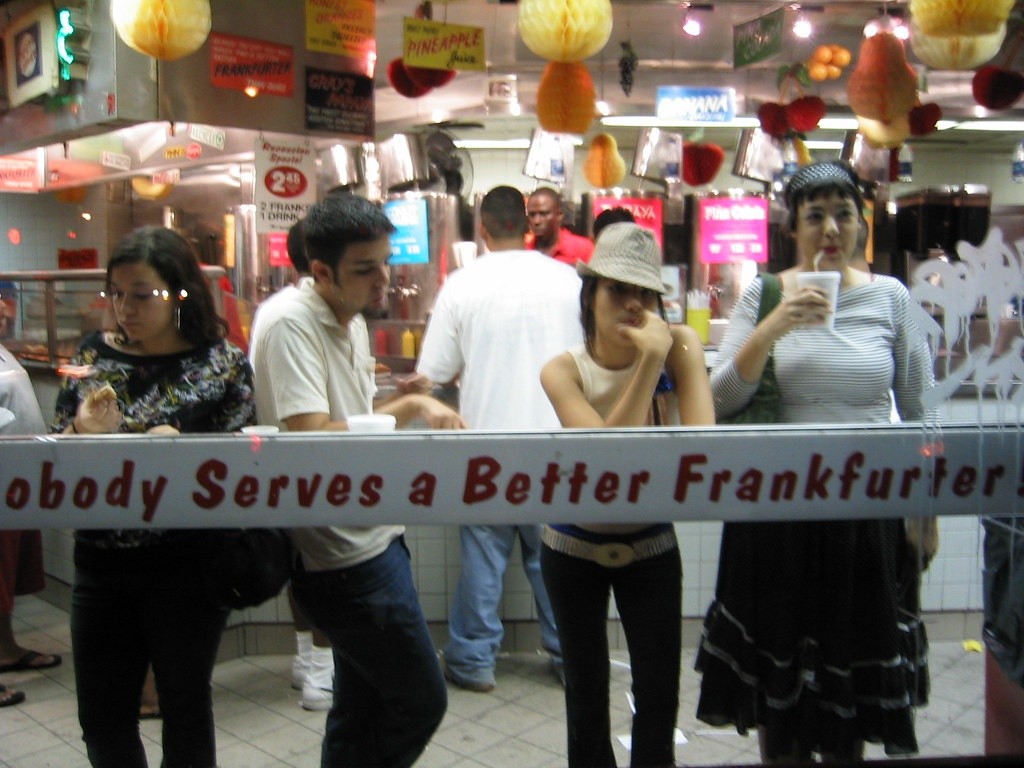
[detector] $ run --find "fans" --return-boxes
[422,130,474,202]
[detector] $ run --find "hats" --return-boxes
[575,221,667,295]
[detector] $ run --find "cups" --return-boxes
[685,308,711,344]
[241,425,280,436]
[797,269,841,327]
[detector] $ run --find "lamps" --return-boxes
[599,47,761,128]
[791,9,813,38]
[953,71,1024,131]
[863,3,906,39]
[815,82,959,130]
[683,7,708,36]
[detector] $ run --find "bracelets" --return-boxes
[72,421,78,433]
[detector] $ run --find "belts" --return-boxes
[539,523,678,565]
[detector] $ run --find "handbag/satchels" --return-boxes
[717,273,781,423]
[199,528,302,613]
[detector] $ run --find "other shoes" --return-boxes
[438,652,493,692]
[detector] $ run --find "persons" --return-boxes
[248,194,466,767]
[528,189,593,263]
[695,159,940,764]
[50,226,256,767]
[595,207,681,327]
[0,281,60,705]
[539,223,713,767]
[415,185,568,692]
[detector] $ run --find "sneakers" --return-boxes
[301,672,334,712]
[290,654,312,690]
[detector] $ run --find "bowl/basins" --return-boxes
[344,411,398,434]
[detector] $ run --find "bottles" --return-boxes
[400,329,416,360]
[372,325,388,355]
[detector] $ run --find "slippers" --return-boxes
[0,683,25,707]
[0,650,62,673]
